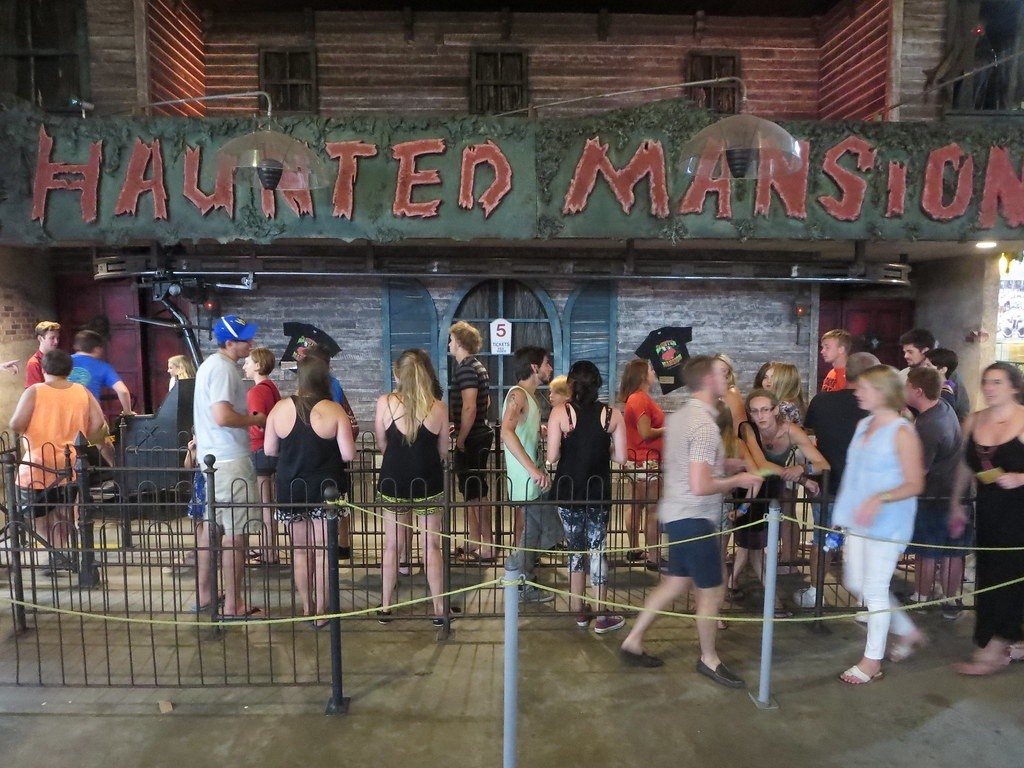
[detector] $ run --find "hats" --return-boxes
[214,315,258,345]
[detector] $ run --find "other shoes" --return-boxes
[792,586,824,609]
[897,592,928,614]
[376,604,391,624]
[696,657,746,689]
[619,648,663,667]
[855,607,871,623]
[943,601,963,619]
[433,606,461,626]
[38,527,980,588]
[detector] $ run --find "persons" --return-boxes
[88,313,110,338]
[167,355,196,392]
[193,314,266,618]
[540,360,625,634]
[375,349,456,627]
[242,348,282,563]
[615,352,765,688]
[9,348,116,576]
[264,355,357,630]
[803,330,970,686]
[447,318,498,562]
[25,321,60,390]
[500,345,565,605]
[297,343,351,560]
[798,328,851,550]
[66,330,137,533]
[183,425,206,558]
[946,362,1024,676]
[618,358,670,570]
[708,353,832,617]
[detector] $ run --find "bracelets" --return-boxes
[879,491,891,503]
[799,462,814,476]
[738,505,748,515]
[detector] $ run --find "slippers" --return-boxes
[192,594,226,611]
[303,611,316,622]
[838,663,884,685]
[218,606,261,617]
[313,610,343,630]
[889,634,928,662]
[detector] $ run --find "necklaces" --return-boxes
[991,405,1018,425]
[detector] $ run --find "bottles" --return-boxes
[823,528,846,551]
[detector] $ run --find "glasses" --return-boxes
[749,405,775,417]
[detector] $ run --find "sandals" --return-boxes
[952,640,1024,675]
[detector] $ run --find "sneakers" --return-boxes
[518,585,554,603]
[595,608,625,634]
[576,603,592,627]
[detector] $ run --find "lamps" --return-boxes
[490,77,757,181]
[98,89,285,189]
